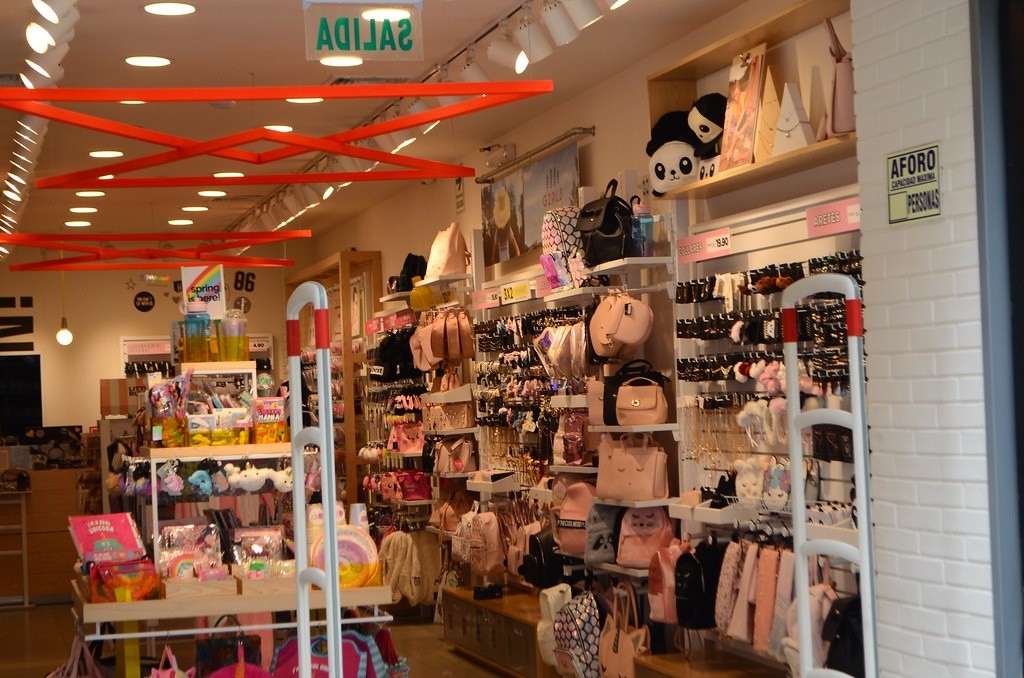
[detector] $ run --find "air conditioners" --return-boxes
[485,144,516,167]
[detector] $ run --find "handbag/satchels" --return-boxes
[602,359,672,426]
[587,379,606,425]
[421,402,446,429]
[424,222,471,280]
[596,430,670,500]
[44,618,414,678]
[428,402,476,431]
[588,295,623,357]
[85,553,162,604]
[421,433,440,473]
[430,434,479,474]
[615,377,668,426]
[405,305,477,394]
[606,295,655,346]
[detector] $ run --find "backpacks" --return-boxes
[396,252,427,292]
[540,204,611,293]
[429,487,473,543]
[451,481,720,678]
[574,179,641,268]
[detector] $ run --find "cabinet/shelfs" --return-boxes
[442,586,560,678]
[466,256,679,577]
[380,275,480,537]
[635,650,790,678]
[69,361,391,678]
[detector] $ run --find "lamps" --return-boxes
[224,0,630,246]
[0,0,81,266]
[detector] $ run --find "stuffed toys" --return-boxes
[644,92,728,197]
[106,459,295,496]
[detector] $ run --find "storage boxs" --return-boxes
[161,578,237,599]
[234,574,297,595]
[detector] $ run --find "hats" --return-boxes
[687,93,728,158]
